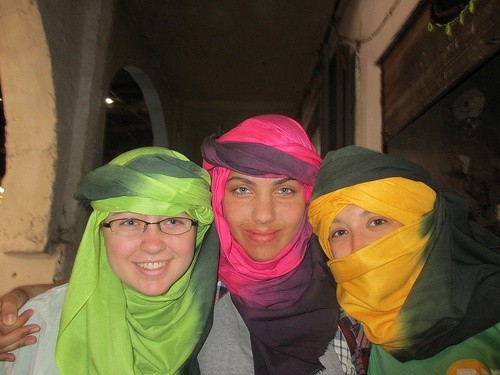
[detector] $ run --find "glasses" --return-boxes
[102,217,197,236]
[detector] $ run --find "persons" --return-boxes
[0,114,500,375]
